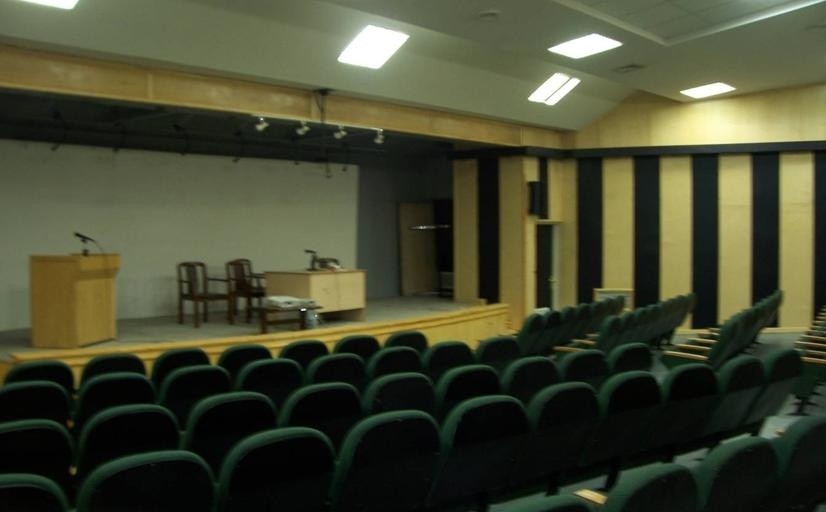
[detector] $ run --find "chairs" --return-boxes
[227,260,265,321]
[177,262,234,328]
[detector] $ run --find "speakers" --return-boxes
[528,182,548,215]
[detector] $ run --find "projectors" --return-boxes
[268,296,298,308]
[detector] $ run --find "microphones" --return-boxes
[73,232,95,243]
[304,249,316,254]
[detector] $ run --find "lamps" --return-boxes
[252,113,385,144]
